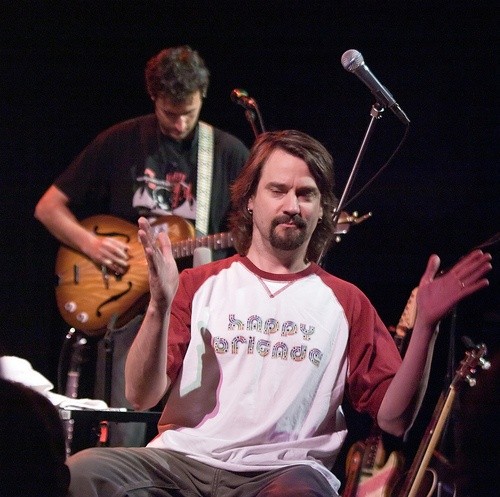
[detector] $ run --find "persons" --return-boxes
[0,377,71,497]
[65,128,493,497]
[34,46,251,447]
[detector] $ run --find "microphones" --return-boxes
[341,48,410,127]
[231,87,256,110]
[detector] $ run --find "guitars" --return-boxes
[341,286,420,497]
[53,208,373,337]
[392,342,490,497]
